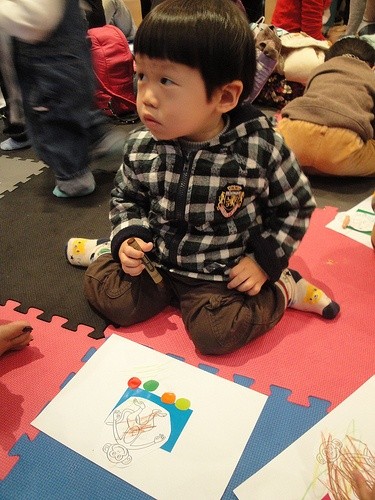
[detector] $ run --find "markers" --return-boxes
[127,237,164,289]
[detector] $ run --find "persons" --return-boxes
[0,321,35,357]
[271,0,375,212]
[66,0,340,356]
[0,0,136,197]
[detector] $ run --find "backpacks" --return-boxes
[87,24,141,124]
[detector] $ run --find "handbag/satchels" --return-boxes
[243,16,279,106]
